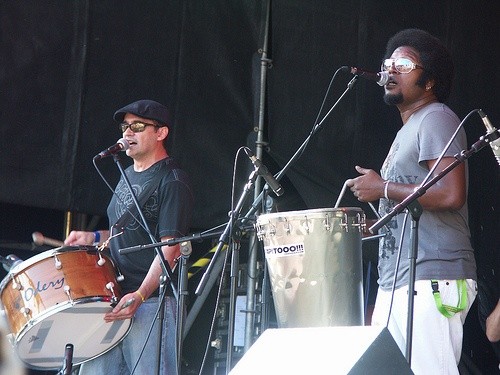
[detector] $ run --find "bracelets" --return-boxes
[383,179,391,200]
[135,290,146,303]
[93,232,101,244]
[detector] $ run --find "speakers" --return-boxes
[228,326,414,375]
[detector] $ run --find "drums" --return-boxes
[255,206,367,329]
[0,244,136,371]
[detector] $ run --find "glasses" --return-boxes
[120,121,170,140]
[381,59,425,74]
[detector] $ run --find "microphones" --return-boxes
[479,109,500,166]
[245,147,285,196]
[94,137,130,160]
[342,66,390,86]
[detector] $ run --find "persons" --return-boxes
[65,99,194,375]
[486,296,500,342]
[344,28,479,375]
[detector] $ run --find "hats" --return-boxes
[113,100,172,128]
